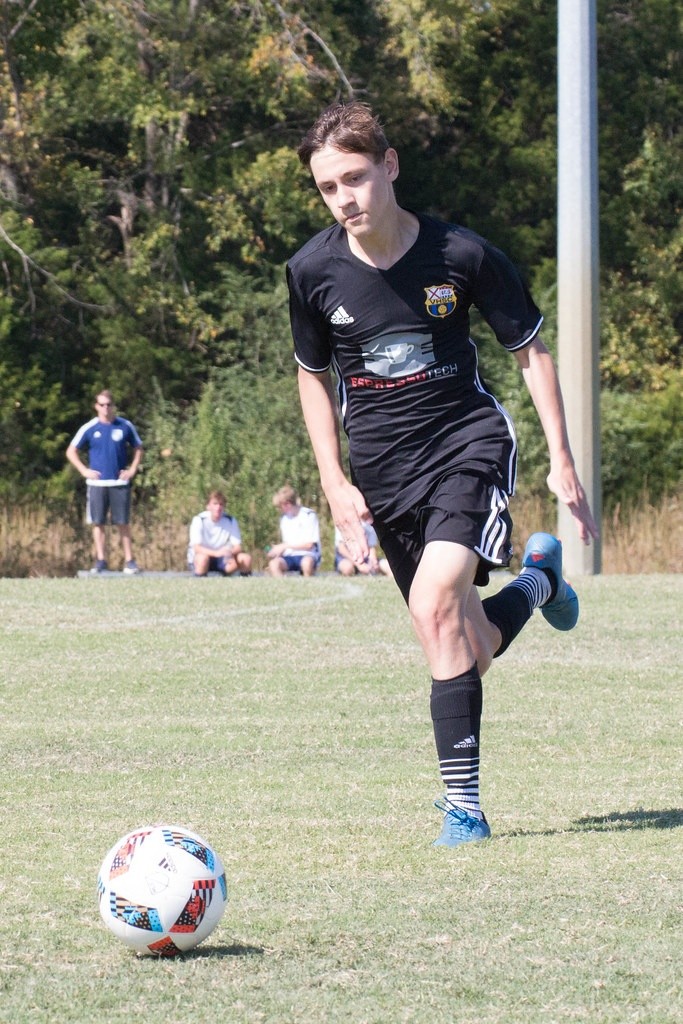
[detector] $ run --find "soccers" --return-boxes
[92,823,232,961]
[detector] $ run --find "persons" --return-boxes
[66,390,143,575]
[267,485,321,576]
[187,490,252,577]
[284,101,599,842]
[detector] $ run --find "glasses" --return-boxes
[97,403,112,407]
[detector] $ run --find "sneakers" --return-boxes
[90,560,108,573]
[123,560,140,573]
[523,533,579,631]
[432,798,492,850]
